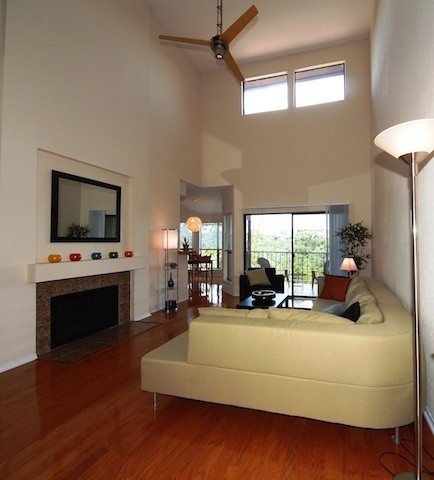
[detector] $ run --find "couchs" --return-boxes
[141,276,427,445]
[239,268,284,302]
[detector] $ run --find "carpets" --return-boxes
[38,320,162,363]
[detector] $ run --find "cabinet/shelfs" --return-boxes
[161,249,179,314]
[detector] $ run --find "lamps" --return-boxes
[373,118,434,480]
[162,226,178,249]
[212,44,226,59]
[340,257,357,278]
[186,193,202,232]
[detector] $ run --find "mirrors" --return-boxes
[51,169,121,242]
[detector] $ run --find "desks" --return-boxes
[188,256,213,284]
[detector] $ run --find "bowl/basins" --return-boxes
[125,251,133,257]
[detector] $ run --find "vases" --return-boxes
[168,272,174,287]
[125,251,133,257]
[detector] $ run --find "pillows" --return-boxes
[245,267,271,287]
[319,276,351,302]
[346,292,383,324]
[344,276,368,307]
[339,302,361,322]
[197,307,268,319]
[320,303,345,316]
[268,308,355,324]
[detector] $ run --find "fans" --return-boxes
[159,0,259,82]
